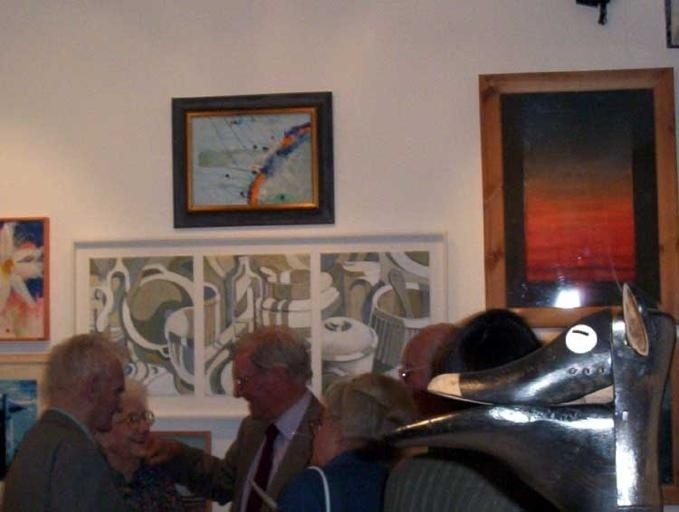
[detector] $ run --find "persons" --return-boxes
[95,377,181,512]
[277,309,556,511]
[2,333,124,511]
[146,324,327,511]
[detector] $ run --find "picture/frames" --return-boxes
[68,228,450,421]
[171,91,335,228]
[479,67,678,328]
[0,353,50,493]
[150,431,212,512]
[0,216,50,341]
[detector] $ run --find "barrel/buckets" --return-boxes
[369,282,428,369]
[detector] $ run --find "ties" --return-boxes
[245,425,280,512]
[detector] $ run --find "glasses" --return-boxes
[235,372,264,387]
[307,417,329,432]
[127,410,155,425]
[398,365,433,380]
